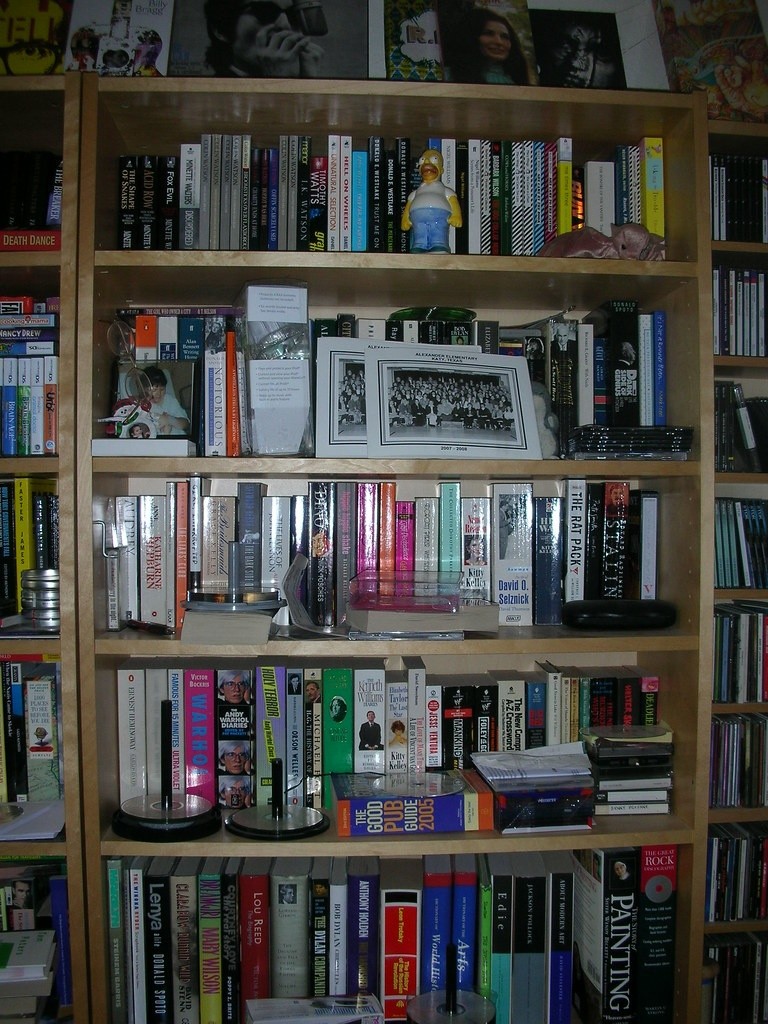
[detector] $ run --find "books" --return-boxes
[527,310,694,461]
[0,148,63,457]
[118,134,664,256]
[92,285,246,459]
[0,479,768,1024]
[707,153,768,472]
[0,0,767,122]
[315,313,542,360]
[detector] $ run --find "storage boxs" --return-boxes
[348,567,463,612]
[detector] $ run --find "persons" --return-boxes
[553,326,576,353]
[338,371,515,431]
[289,673,301,695]
[206,321,225,349]
[314,881,327,895]
[455,9,529,85]
[13,880,33,910]
[278,884,297,904]
[219,742,255,776]
[359,711,383,750]
[305,682,322,703]
[204,0,323,79]
[218,671,254,705]
[528,338,544,360]
[389,721,407,747]
[136,367,191,434]
[220,777,253,808]
[617,342,635,365]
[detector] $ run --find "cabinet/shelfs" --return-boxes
[77,74,711,1023]
[0,69,90,1024]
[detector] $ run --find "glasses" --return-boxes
[226,681,248,691]
[226,752,249,761]
[227,787,249,795]
[240,2,302,30]
